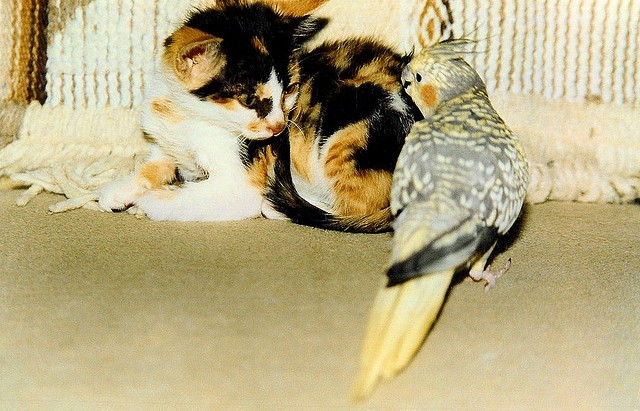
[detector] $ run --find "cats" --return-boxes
[98,0,425,233]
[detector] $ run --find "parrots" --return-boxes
[349,21,532,408]
